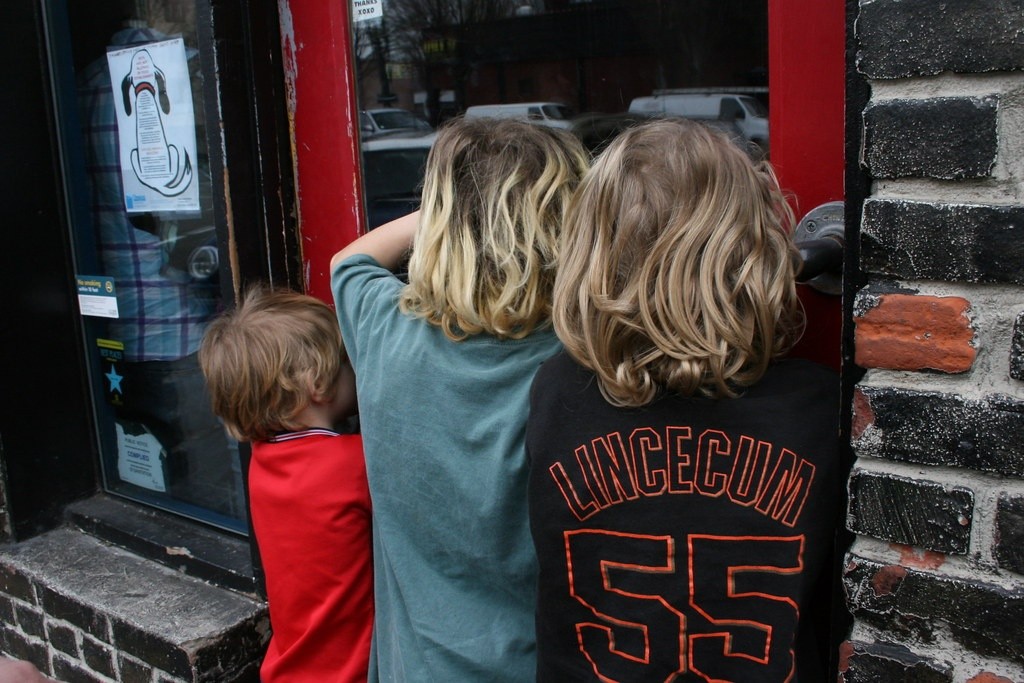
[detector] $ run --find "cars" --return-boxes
[360,108,433,142]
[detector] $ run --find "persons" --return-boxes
[72,0,238,518]
[523,118,855,683]
[325,118,593,683]
[199,289,374,683]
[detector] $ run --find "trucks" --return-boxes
[630,94,769,147]
[464,102,574,129]
